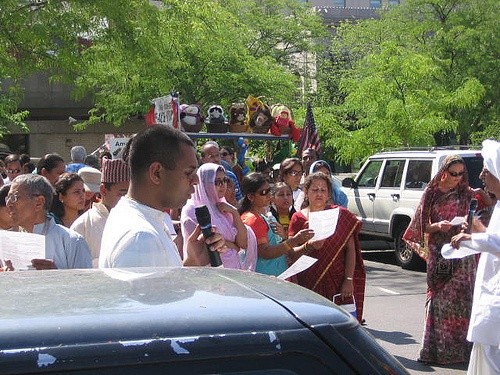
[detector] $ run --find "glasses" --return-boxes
[255,187,271,196]
[287,170,306,176]
[5,193,45,203]
[447,169,464,177]
[215,176,230,186]
[5,167,23,174]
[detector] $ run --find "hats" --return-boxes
[100,159,131,182]
[481,139,500,183]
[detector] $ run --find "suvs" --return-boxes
[335,144,484,270]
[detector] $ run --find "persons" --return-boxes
[406,137,500,375]
[0,123,367,324]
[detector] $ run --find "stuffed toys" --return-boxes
[178,94,303,141]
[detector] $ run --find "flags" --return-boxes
[297,103,323,157]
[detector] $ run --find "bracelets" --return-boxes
[303,242,310,253]
[283,241,291,251]
[344,276,354,280]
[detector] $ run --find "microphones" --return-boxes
[194,204,222,267]
[463,198,478,234]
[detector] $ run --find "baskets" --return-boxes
[205,119,229,133]
[230,122,249,132]
[271,104,295,134]
[250,121,272,133]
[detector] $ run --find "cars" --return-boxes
[0,266,412,375]
